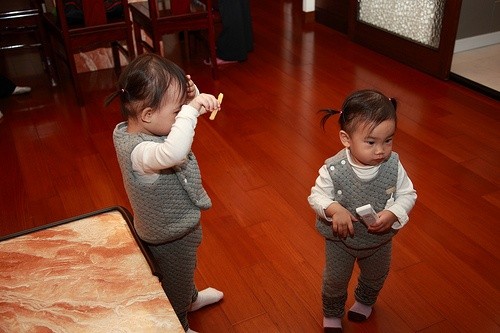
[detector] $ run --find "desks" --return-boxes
[0,205,187,333]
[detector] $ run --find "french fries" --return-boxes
[209,93,223,120]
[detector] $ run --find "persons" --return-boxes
[106,55,223,333]
[204,0,254,65]
[307,89,418,333]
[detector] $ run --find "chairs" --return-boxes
[44,0,136,106]
[129,0,220,80]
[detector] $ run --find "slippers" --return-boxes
[204,57,239,65]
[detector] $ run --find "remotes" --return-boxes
[356,204,380,227]
